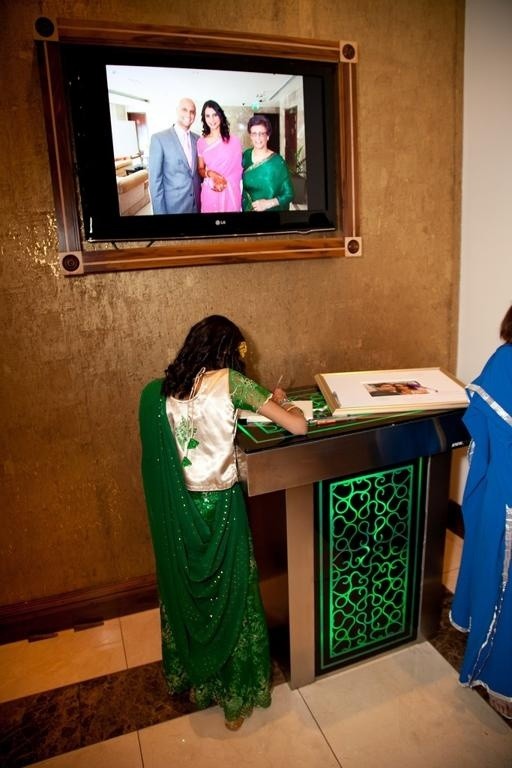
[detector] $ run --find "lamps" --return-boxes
[127,112,146,157]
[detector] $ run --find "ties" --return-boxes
[184,133,192,168]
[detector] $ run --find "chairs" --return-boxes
[114,156,133,177]
[115,169,150,217]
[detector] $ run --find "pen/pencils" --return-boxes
[276,374,284,389]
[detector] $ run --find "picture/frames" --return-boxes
[32,16,363,279]
[314,365,471,418]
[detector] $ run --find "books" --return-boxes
[238,401,313,423]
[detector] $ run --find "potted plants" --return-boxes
[281,145,308,205]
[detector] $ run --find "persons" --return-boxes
[146,97,202,214]
[137,315,309,732]
[241,115,296,211]
[369,383,400,397]
[196,99,245,213]
[393,383,427,393]
[448,306,512,720]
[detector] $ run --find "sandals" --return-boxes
[488,693,512,719]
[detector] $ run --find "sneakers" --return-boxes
[188,686,253,731]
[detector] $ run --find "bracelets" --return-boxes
[279,398,299,412]
[204,169,212,178]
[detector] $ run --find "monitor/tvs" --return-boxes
[56,34,340,241]
